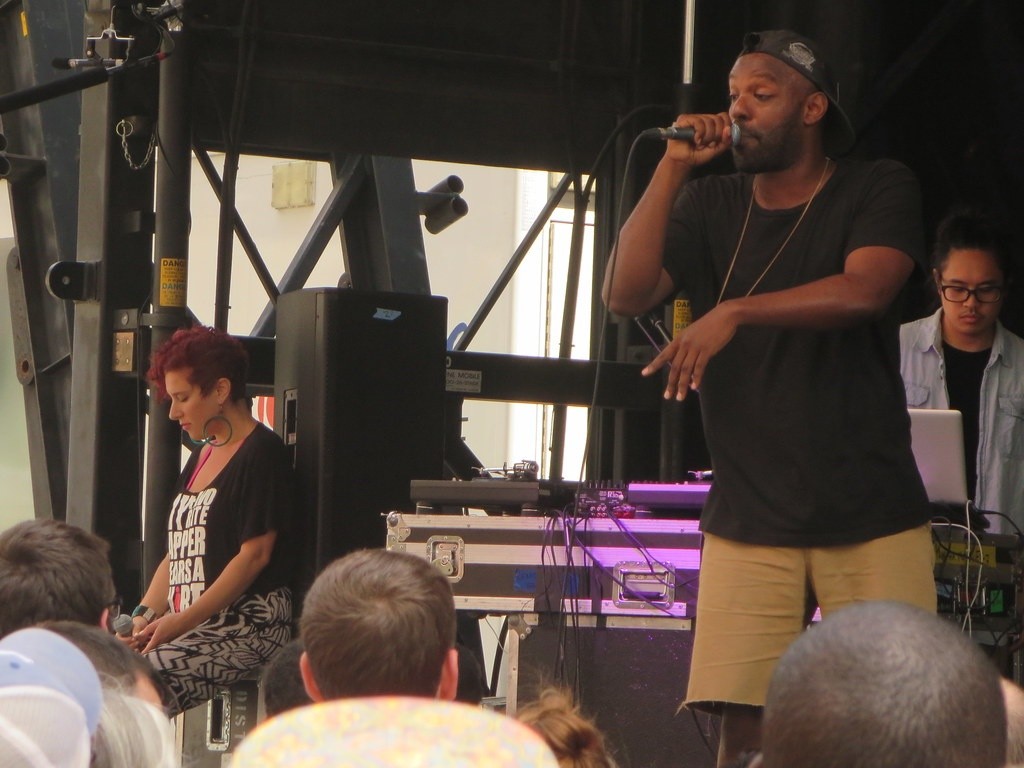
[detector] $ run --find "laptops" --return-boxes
[907,408,990,529]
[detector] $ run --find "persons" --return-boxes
[227,549,561,768]
[0,620,171,768]
[0,518,116,639]
[518,689,618,768]
[454,644,483,705]
[262,641,316,718]
[899,214,1024,536]
[600,30,938,768]
[115,326,295,719]
[761,601,1007,768]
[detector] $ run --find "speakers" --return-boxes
[276,287,449,637]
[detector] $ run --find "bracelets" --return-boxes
[132,606,158,625]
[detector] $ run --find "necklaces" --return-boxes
[717,156,831,304]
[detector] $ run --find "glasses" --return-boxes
[100,597,122,621]
[937,270,1009,303]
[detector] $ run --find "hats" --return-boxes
[0,627,101,767]
[736,29,854,159]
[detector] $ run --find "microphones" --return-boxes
[645,124,741,147]
[114,614,147,654]
